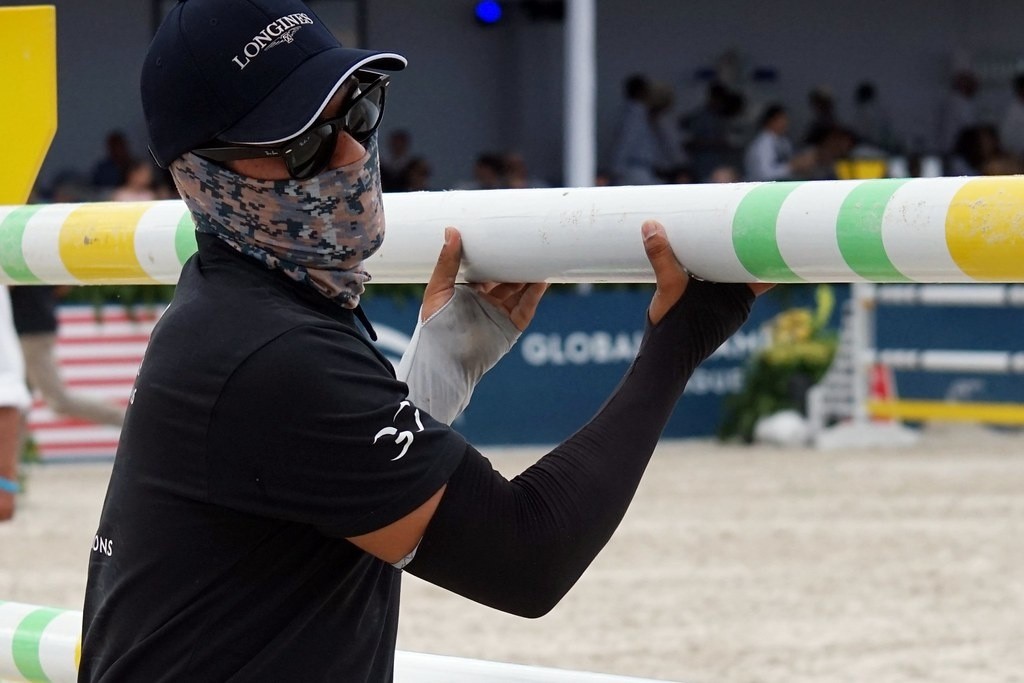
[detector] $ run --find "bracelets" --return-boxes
[0,475,24,497]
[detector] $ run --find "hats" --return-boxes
[141,0,409,168]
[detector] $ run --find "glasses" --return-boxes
[192,68,390,181]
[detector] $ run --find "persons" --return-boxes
[1,265,32,524]
[81,0,777,683]
[4,32,1024,340]
[6,282,128,427]
